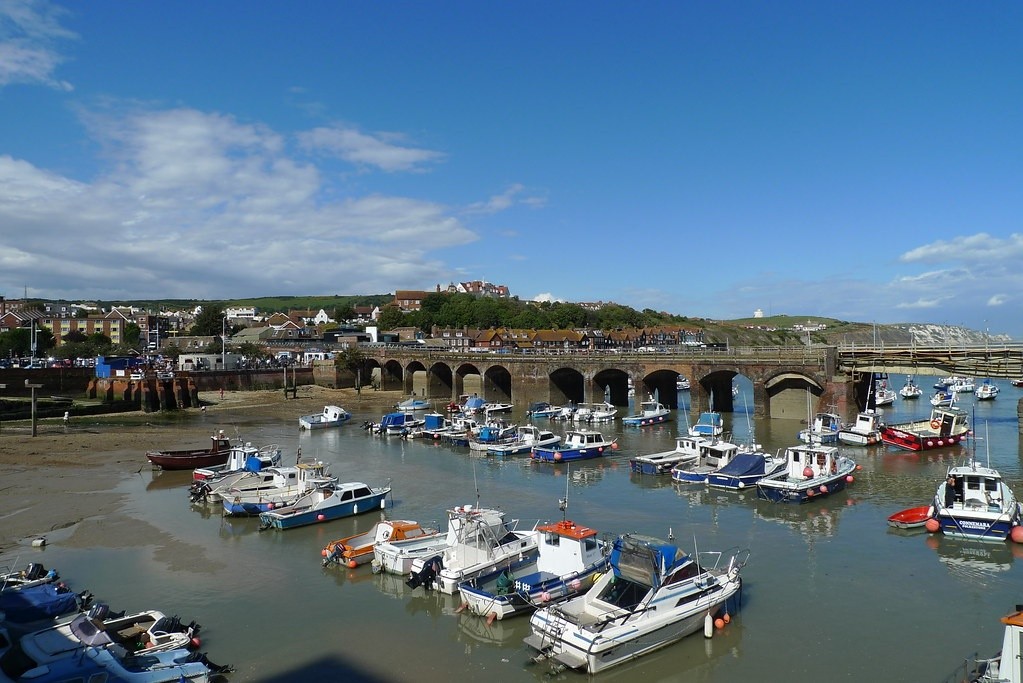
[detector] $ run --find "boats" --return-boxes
[732,378,740,398]
[0,555,237,683]
[796,360,1022,451]
[621,385,861,506]
[322,462,749,676]
[360,395,618,464]
[676,375,690,389]
[145,417,392,531]
[298,405,351,430]
[887,405,1021,544]
[940,604,1023,683]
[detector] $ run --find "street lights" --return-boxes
[872,319,875,350]
[223,316,226,370]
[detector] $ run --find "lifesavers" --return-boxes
[557,520,575,528]
[931,420,939,429]
[831,460,836,472]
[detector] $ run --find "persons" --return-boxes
[945,478,956,508]
[220,388,223,400]
